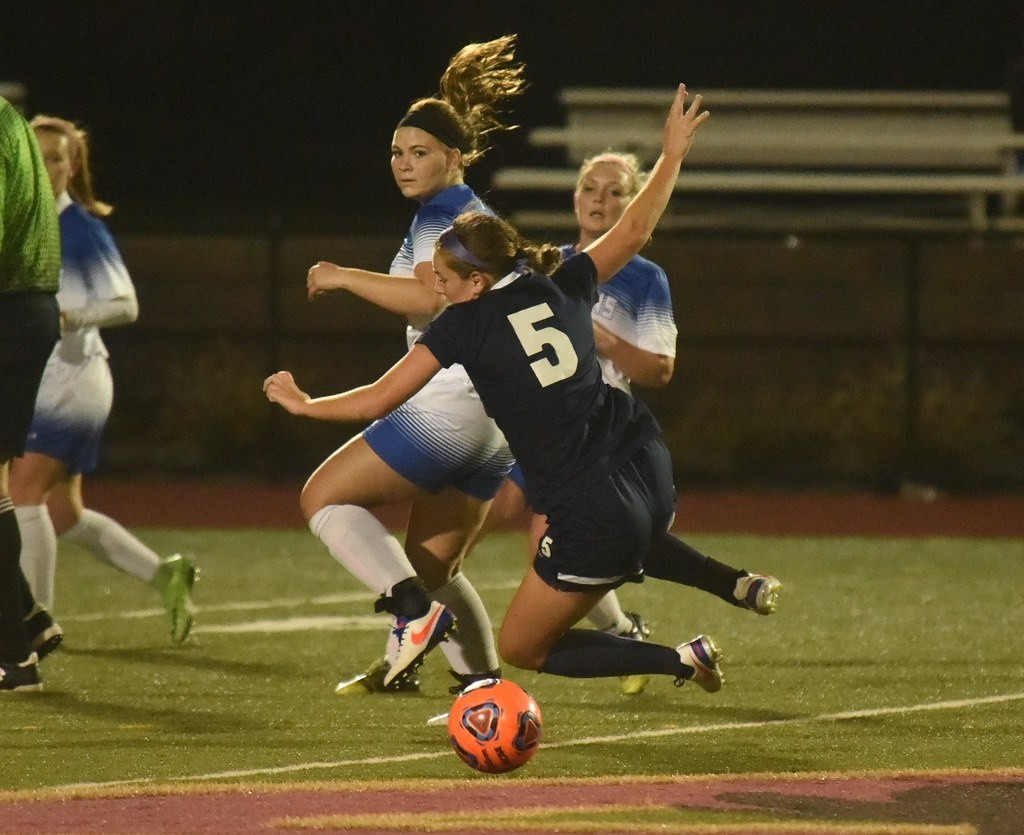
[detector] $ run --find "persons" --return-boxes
[7,113,201,651]
[261,77,782,692]
[335,147,679,695]
[0,85,64,696]
[299,26,522,729]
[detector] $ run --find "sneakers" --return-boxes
[382,601,456,689]
[0,651,42,693]
[152,551,201,646]
[426,711,451,726]
[20,603,62,662]
[334,657,422,693]
[732,573,782,615]
[677,635,725,694]
[597,612,650,696]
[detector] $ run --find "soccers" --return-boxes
[450,679,541,773]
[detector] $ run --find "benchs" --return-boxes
[487,87,1023,238]
